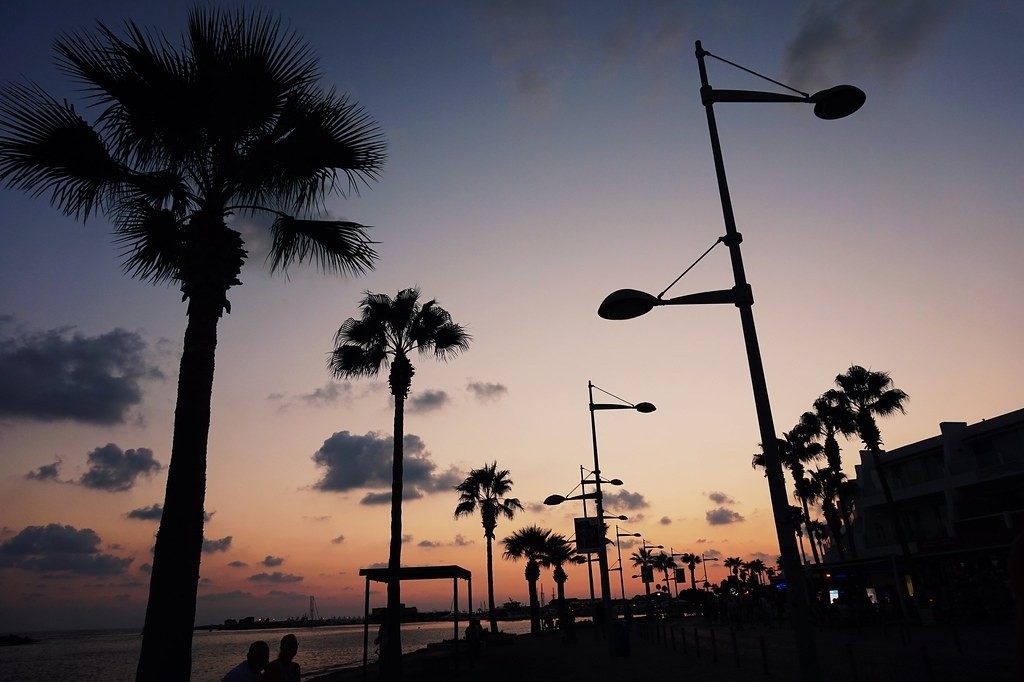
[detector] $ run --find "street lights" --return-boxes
[544,379,716,655]
[597,40,866,676]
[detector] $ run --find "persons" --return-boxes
[374,609,386,645]
[221,634,301,682]
[559,626,566,645]
[816,608,825,632]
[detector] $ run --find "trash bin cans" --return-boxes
[607,622,631,657]
